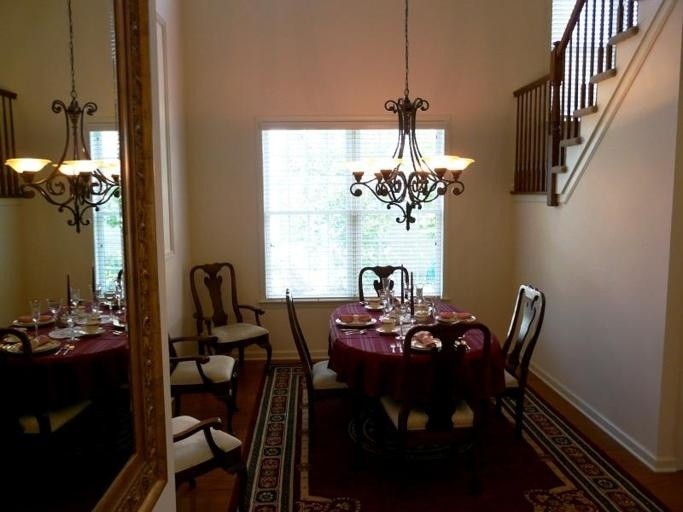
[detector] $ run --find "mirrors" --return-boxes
[0,0,168,509]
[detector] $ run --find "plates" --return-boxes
[411,339,442,351]
[3,335,36,343]
[334,314,377,327]
[365,304,384,311]
[434,311,476,324]
[12,313,54,329]
[6,340,62,355]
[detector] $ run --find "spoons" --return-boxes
[344,329,367,336]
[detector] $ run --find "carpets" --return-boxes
[230,358,675,511]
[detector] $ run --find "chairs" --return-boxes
[2,325,101,485]
[171,396,250,512]
[375,315,490,497]
[488,285,546,439]
[188,264,274,379]
[168,325,242,435]
[282,286,366,439]
[357,264,410,304]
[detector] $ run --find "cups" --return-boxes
[366,298,380,308]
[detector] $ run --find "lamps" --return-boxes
[347,0,476,232]
[7,0,122,233]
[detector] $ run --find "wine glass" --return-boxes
[375,277,431,341]
[28,286,116,341]
